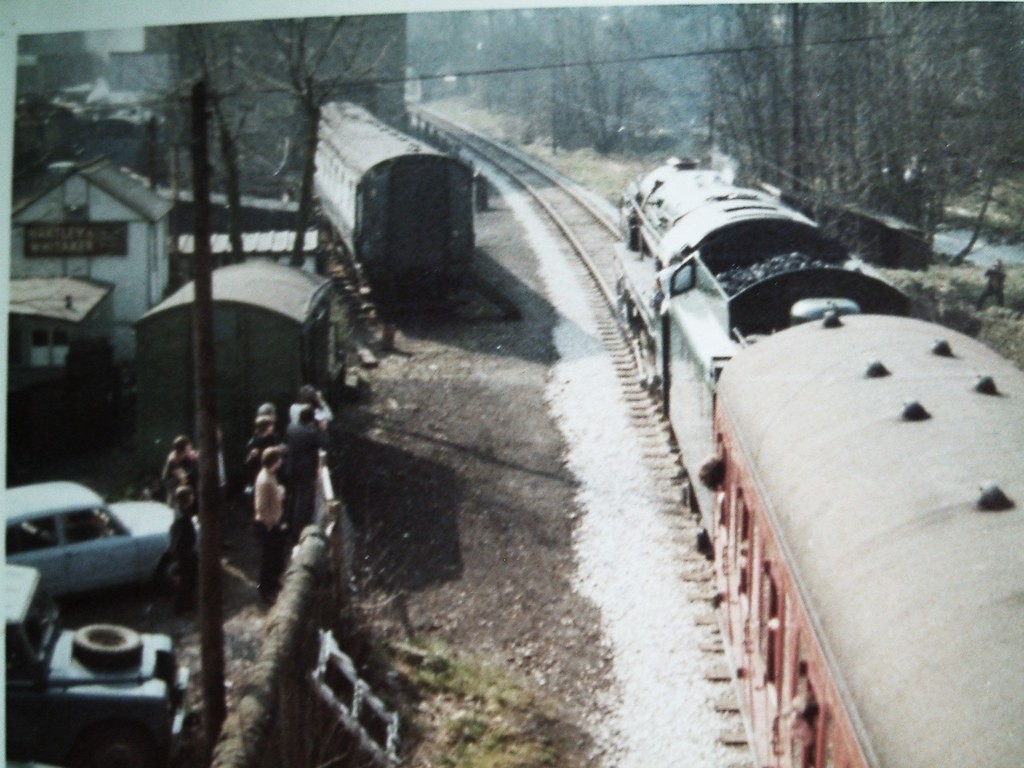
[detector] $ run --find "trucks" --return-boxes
[3,563,184,767]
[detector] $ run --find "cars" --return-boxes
[3,481,200,602]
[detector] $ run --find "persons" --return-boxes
[241,385,333,610]
[159,427,238,613]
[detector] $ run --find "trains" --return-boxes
[617,161,1023,765]
[307,98,481,307]
